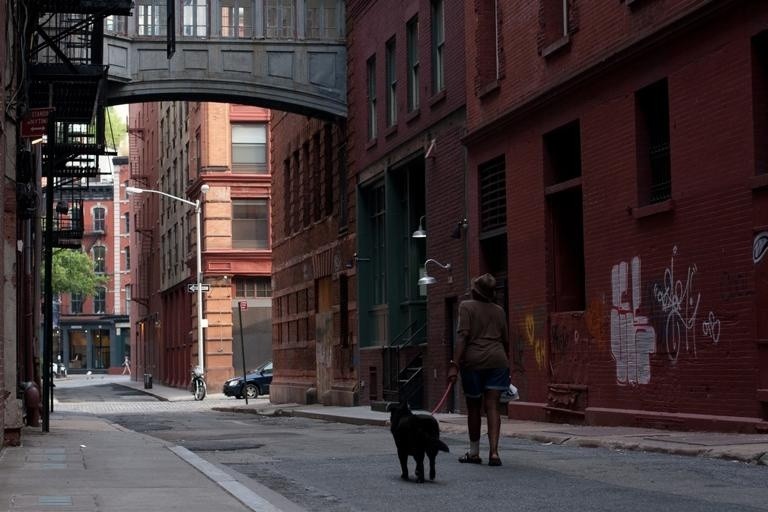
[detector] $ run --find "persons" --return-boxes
[121,355,132,375]
[445,271,512,466]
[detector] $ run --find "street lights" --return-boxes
[125,183,205,380]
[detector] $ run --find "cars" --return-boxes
[222,360,274,400]
[49,362,67,379]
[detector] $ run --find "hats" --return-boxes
[471,272,497,302]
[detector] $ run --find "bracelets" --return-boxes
[448,358,460,367]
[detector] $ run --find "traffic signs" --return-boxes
[188,283,209,292]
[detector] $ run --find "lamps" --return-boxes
[413,215,427,238]
[418,259,451,286]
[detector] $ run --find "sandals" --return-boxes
[458,452,482,463]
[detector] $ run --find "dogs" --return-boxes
[384,400,449,483]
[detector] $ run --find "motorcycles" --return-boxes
[188,363,209,401]
